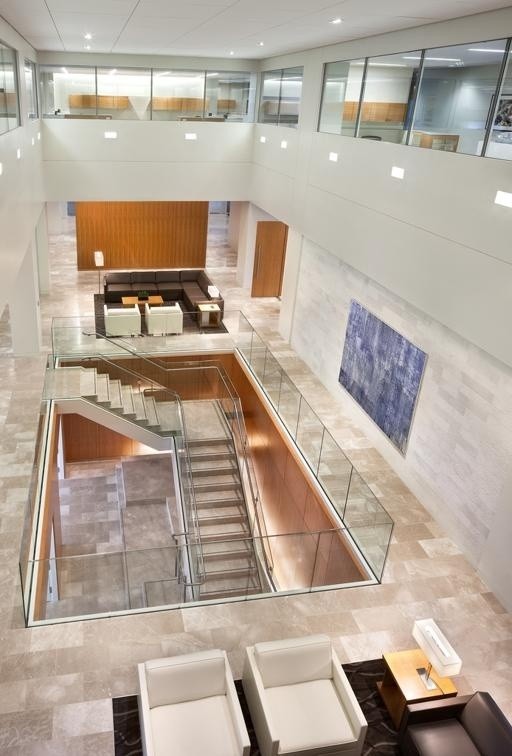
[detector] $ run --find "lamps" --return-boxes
[95,251,105,295]
[411,618,463,690]
[207,285,220,308]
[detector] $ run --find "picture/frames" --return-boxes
[484,93,512,132]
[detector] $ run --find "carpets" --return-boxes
[112,660,401,756]
[94,294,229,339]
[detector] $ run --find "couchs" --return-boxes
[105,269,225,322]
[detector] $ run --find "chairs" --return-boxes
[104,302,183,337]
[396,692,511,756]
[135,633,367,755]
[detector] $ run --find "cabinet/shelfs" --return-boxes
[151,97,236,110]
[342,100,408,123]
[68,94,129,109]
[375,647,458,731]
[197,303,221,328]
